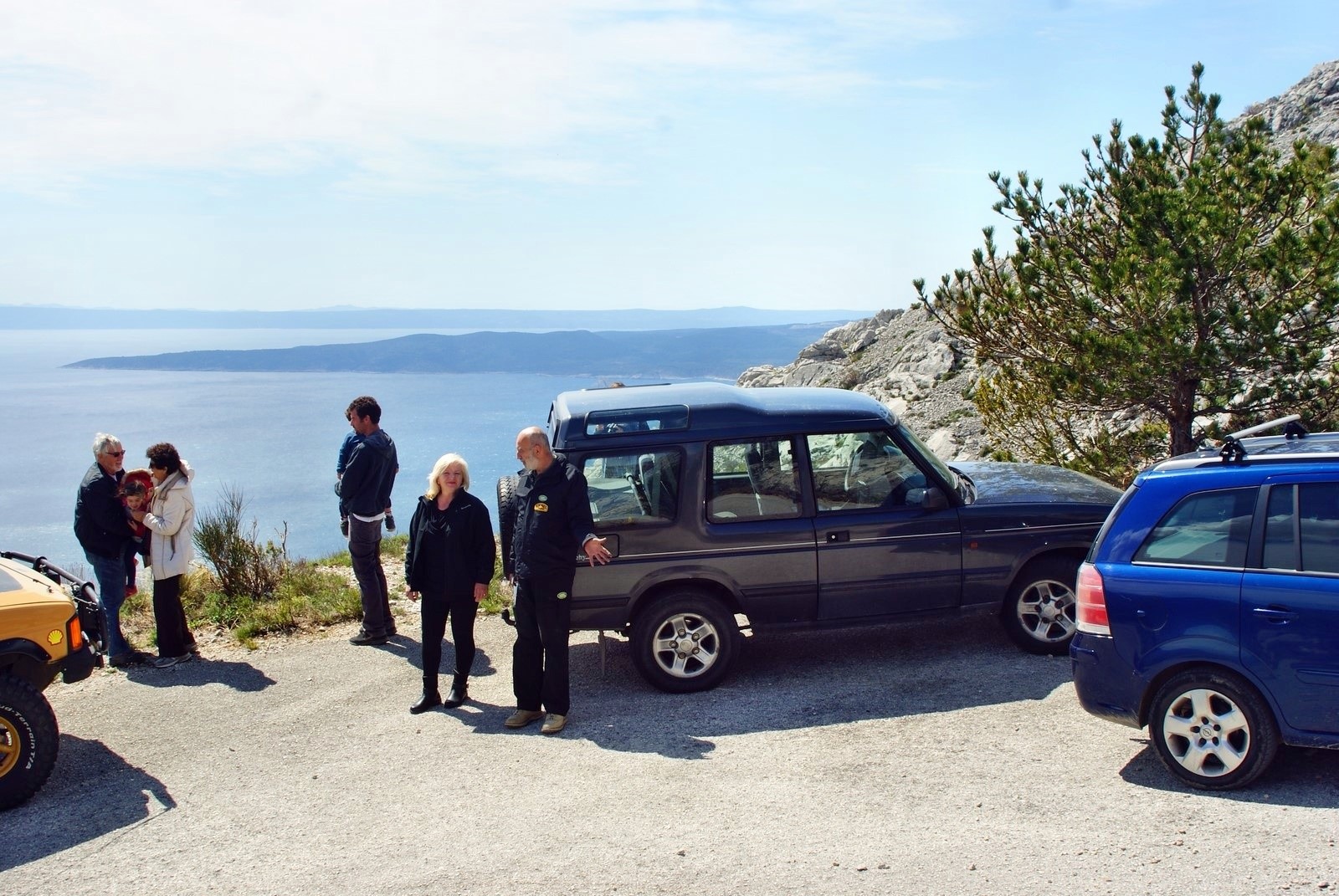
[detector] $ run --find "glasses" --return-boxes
[106,450,126,457]
[150,462,155,468]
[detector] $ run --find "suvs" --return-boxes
[0,551,108,813]
[496,383,1125,694]
[1071,410,1339,791]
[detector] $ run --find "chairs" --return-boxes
[642,468,677,518]
[745,446,768,515]
[756,480,803,514]
[638,454,655,486]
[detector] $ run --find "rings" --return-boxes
[483,593,487,596]
[134,514,137,517]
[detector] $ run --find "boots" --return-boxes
[444,668,469,708]
[410,675,441,715]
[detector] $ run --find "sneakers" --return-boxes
[504,708,543,727]
[540,714,568,732]
[155,642,198,668]
[384,514,396,532]
[350,623,399,644]
[125,585,138,600]
[340,518,349,539]
[134,558,138,566]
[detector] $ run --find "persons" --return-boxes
[505,426,613,735]
[336,399,402,537]
[405,451,497,712]
[129,441,199,668]
[118,473,150,600]
[334,394,398,646]
[73,434,149,663]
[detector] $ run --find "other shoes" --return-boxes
[111,650,133,667]
